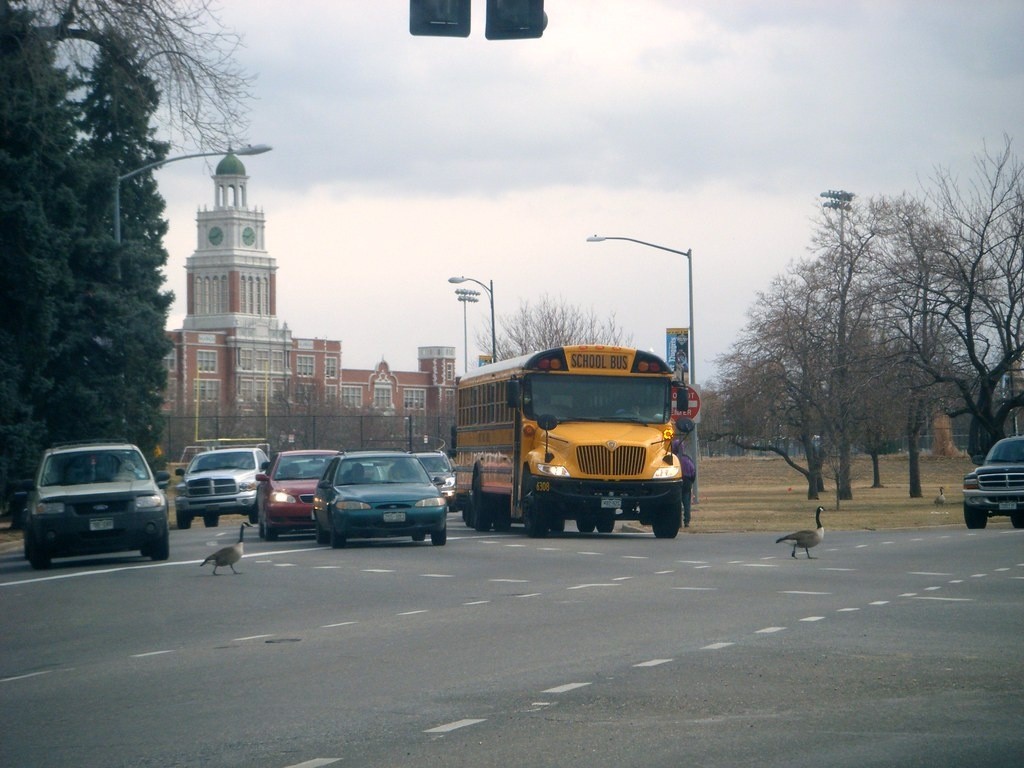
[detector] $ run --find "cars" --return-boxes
[24,443,170,570]
[11,479,33,529]
[255,449,341,542]
[313,449,449,549]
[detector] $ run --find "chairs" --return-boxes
[280,464,302,479]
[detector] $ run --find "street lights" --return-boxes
[822,189,856,286]
[454,286,483,374]
[448,275,498,365]
[586,234,699,505]
[115,145,278,281]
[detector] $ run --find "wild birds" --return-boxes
[200,521,253,575]
[776,505,826,559]
[934,487,945,508]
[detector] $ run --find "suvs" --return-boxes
[962,435,1024,530]
[175,446,270,529]
[387,450,459,513]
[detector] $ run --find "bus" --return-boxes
[452,343,695,539]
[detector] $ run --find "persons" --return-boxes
[287,463,300,477]
[350,463,372,482]
[672,440,696,528]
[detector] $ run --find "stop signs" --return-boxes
[668,383,700,420]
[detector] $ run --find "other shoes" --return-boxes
[684,524,689,527]
